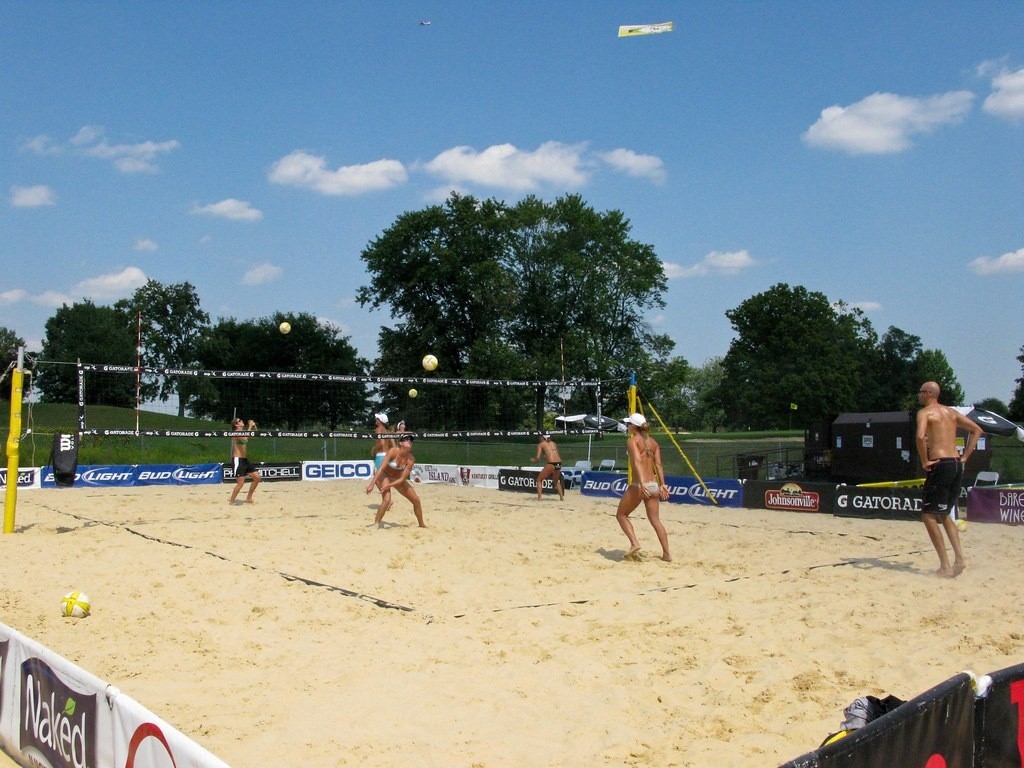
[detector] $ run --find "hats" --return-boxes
[375,413,388,423]
[623,413,645,427]
[397,433,414,441]
[397,420,405,430]
[540,430,550,437]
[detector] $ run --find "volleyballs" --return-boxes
[61,591,90,616]
[409,388,418,398]
[279,321,291,335]
[422,355,438,370]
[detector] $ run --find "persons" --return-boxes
[229,418,260,505]
[804,447,824,481]
[914,381,983,579]
[615,413,672,563]
[366,414,428,529]
[530,429,564,501]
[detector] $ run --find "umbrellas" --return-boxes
[945,405,1024,486]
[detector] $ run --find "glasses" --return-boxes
[918,390,932,393]
[237,419,241,423]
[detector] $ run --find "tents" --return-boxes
[555,414,626,433]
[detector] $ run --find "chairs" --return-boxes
[570,461,591,490]
[974,472,999,486]
[598,459,616,471]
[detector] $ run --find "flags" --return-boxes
[791,402,798,410]
